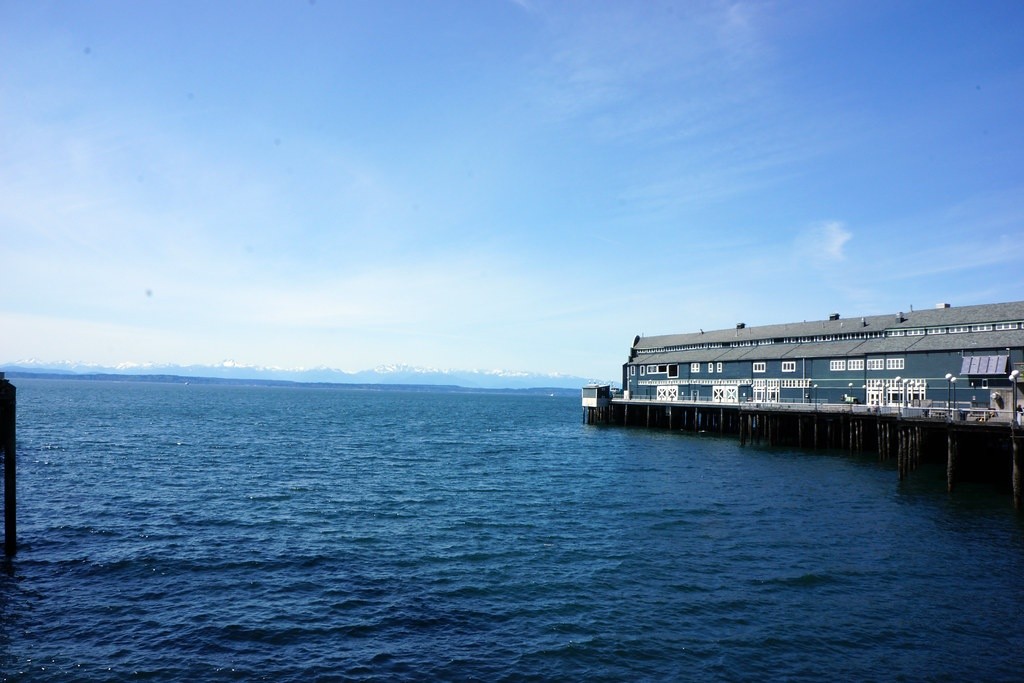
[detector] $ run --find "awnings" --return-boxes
[959,355,1010,376]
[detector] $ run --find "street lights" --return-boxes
[895,376,902,416]
[1009,375,1015,421]
[950,377,958,417]
[849,383,853,405]
[902,377,928,404]
[1011,370,1019,421]
[945,373,953,416]
[813,384,818,403]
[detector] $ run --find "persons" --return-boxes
[1016,405,1023,426]
